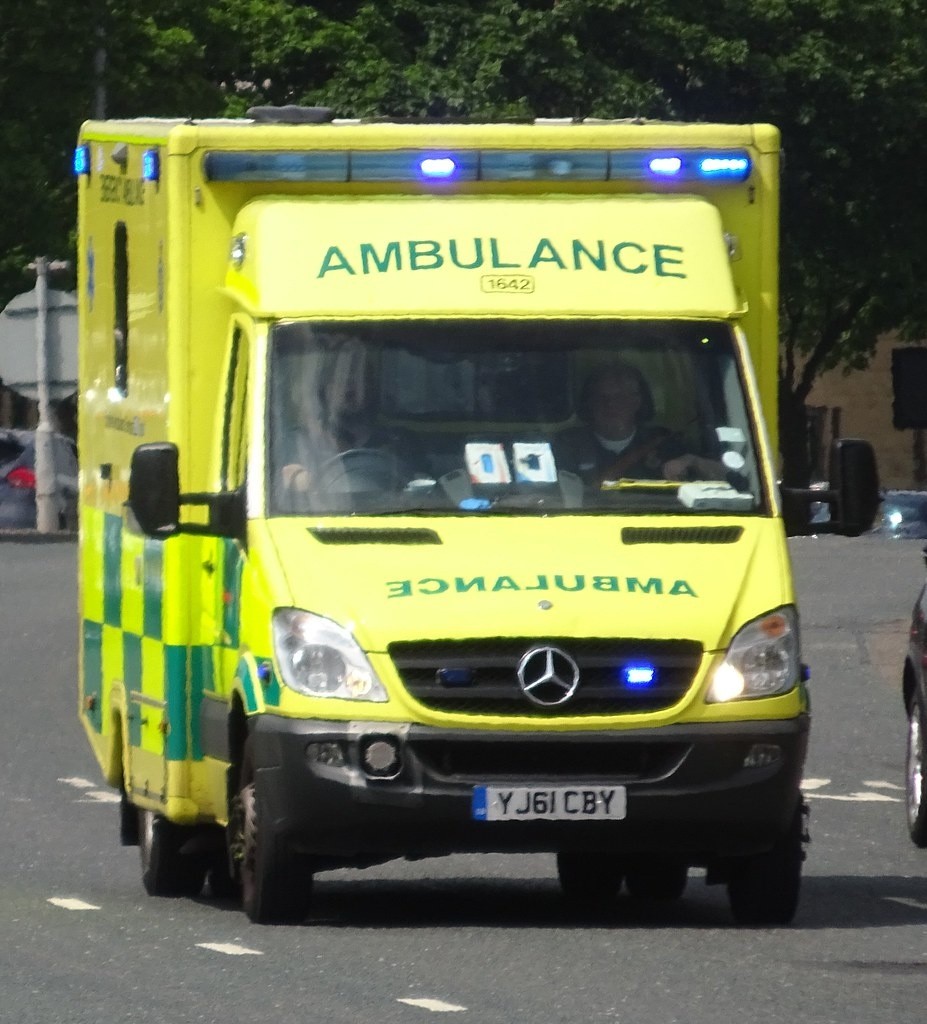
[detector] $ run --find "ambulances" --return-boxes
[72,107,881,923]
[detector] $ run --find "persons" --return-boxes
[551,364,729,488]
[280,352,446,498]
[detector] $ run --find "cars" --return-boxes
[0,427,80,530]
[880,488,927,541]
[900,546,927,849]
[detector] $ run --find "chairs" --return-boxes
[273,421,436,491]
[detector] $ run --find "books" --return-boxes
[464,443,559,486]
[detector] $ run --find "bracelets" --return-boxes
[289,469,305,489]
